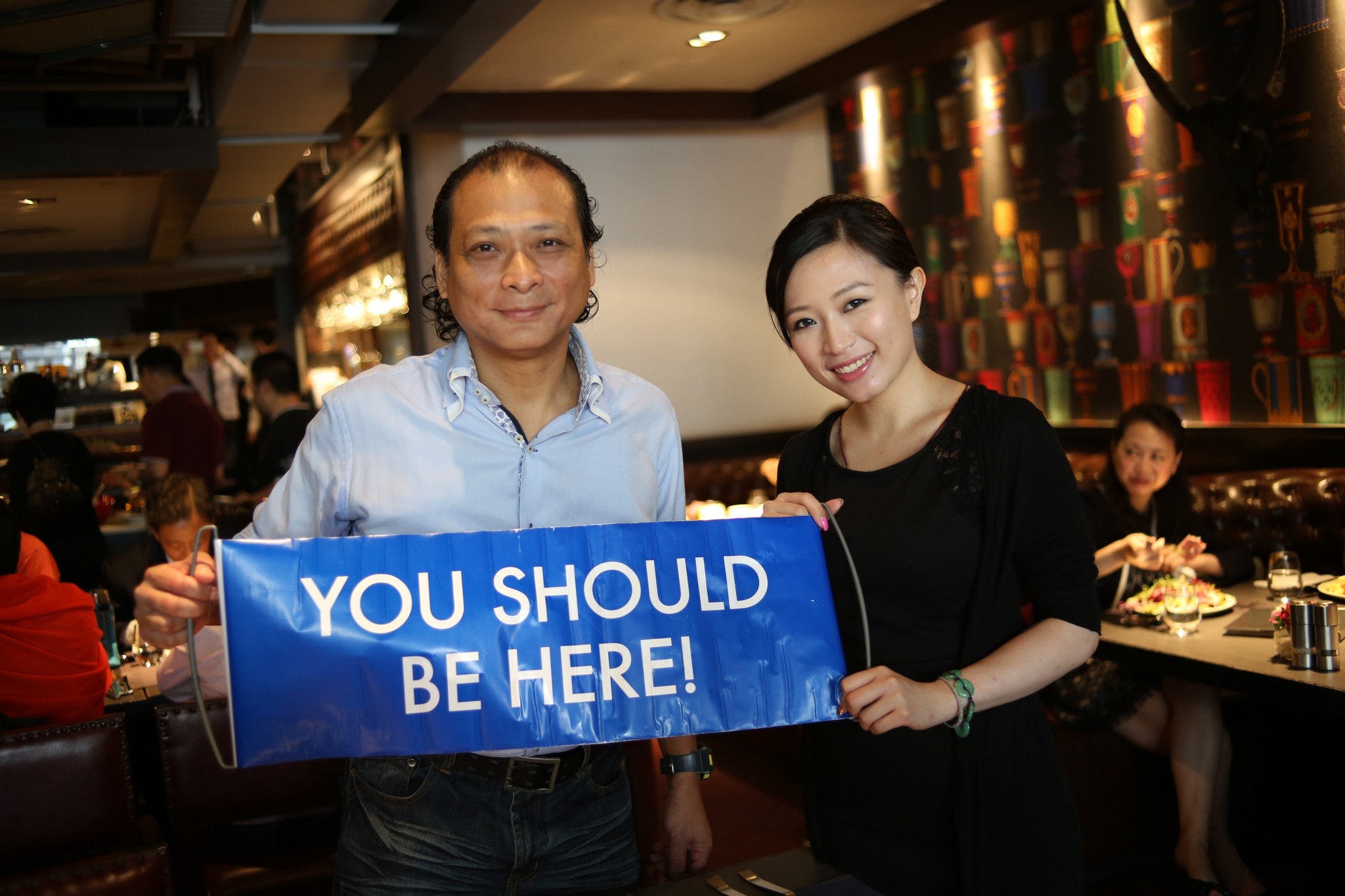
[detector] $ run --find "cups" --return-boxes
[1268,551,1303,601]
[132,624,163,666]
[1163,587,1201,633]
[843,0,1345,424]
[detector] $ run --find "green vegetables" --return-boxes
[1129,576,1225,613]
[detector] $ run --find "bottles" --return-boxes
[1288,599,1340,670]
[90,588,121,669]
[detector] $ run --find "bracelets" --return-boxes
[942,669,975,737]
[937,677,963,728]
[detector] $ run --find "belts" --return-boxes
[423,743,611,795]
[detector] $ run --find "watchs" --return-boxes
[659,746,715,780]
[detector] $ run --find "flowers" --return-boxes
[1270,601,1293,633]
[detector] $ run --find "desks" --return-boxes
[1093,572,1345,896]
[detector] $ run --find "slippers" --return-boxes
[1169,854,1227,895]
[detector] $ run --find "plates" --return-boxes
[1126,593,1235,613]
[1318,579,1345,599]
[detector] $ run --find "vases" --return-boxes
[1273,629,1293,657]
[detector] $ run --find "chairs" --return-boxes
[1021,593,1137,882]
[0,698,342,896]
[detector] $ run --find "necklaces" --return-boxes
[839,383,967,468]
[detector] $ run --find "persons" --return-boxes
[135,142,715,896]
[102,344,223,587]
[0,372,108,595]
[1032,402,1235,896]
[88,334,254,475]
[115,472,240,654]
[223,351,316,492]
[766,195,1102,896]
